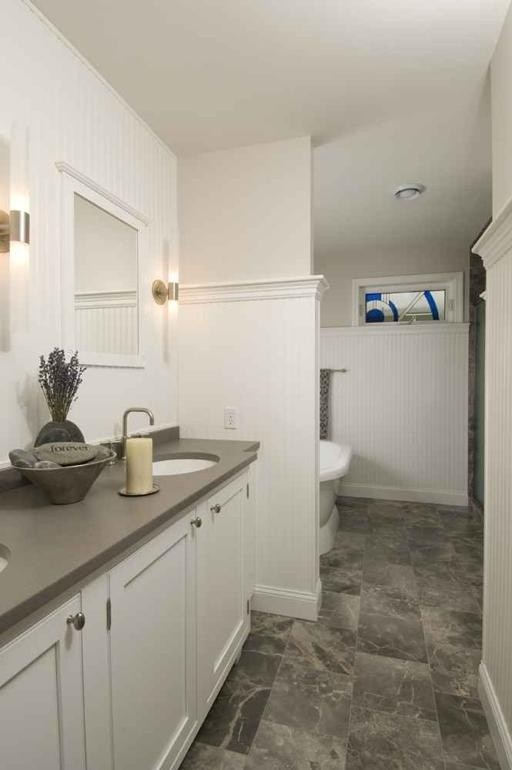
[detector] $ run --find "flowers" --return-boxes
[38,347,87,422]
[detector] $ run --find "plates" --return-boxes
[34,442,98,465]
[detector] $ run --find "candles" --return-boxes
[125,437,153,494]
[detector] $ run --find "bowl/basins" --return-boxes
[8,441,118,505]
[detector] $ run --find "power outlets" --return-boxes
[224,409,237,429]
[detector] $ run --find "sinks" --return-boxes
[152,452,221,476]
[0,541,11,572]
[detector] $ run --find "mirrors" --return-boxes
[59,163,149,368]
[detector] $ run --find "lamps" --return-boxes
[152,258,182,324]
[0,179,34,278]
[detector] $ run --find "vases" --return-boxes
[35,419,87,446]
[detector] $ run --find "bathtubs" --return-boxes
[319,439,353,556]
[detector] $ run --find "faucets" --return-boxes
[122,408,154,461]
